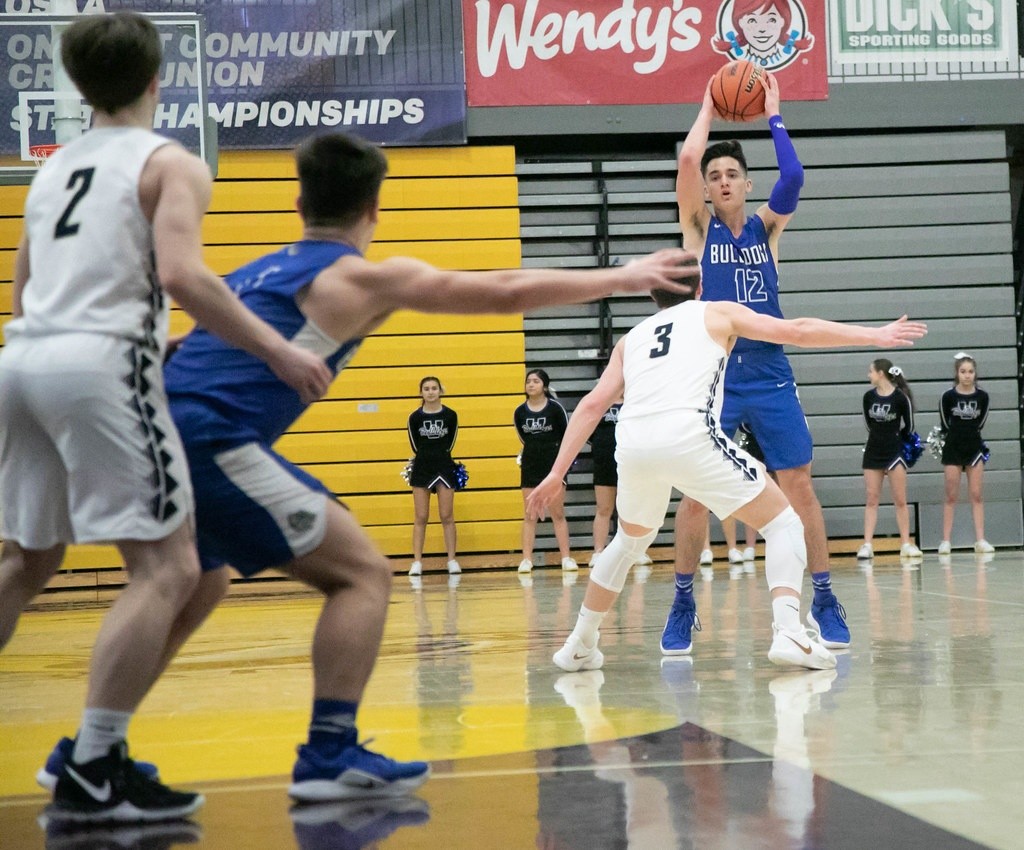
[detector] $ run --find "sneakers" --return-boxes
[562,556,578,571]
[35,737,156,789]
[293,797,431,849]
[661,591,702,654]
[661,656,694,686]
[562,570,579,586]
[634,551,653,565]
[287,735,431,800]
[701,561,756,581]
[410,576,422,588]
[408,560,423,578]
[449,575,460,588]
[46,740,201,824]
[859,553,995,569]
[518,575,532,585]
[769,670,838,698]
[742,548,755,560]
[588,551,602,566]
[552,629,605,671]
[555,670,606,707]
[768,625,836,670]
[633,567,653,583]
[938,540,995,555]
[806,595,851,646]
[698,549,744,564]
[445,560,461,575]
[518,559,532,574]
[856,542,922,558]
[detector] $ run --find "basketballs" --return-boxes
[710,60,771,122]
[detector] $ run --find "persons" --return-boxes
[659,69,850,655]
[519,248,930,674]
[938,353,995,554]
[700,514,744,565]
[740,423,776,560]
[857,359,924,558]
[37,132,699,804]
[0,13,335,823]
[513,369,578,573]
[406,377,462,576]
[585,363,655,566]
